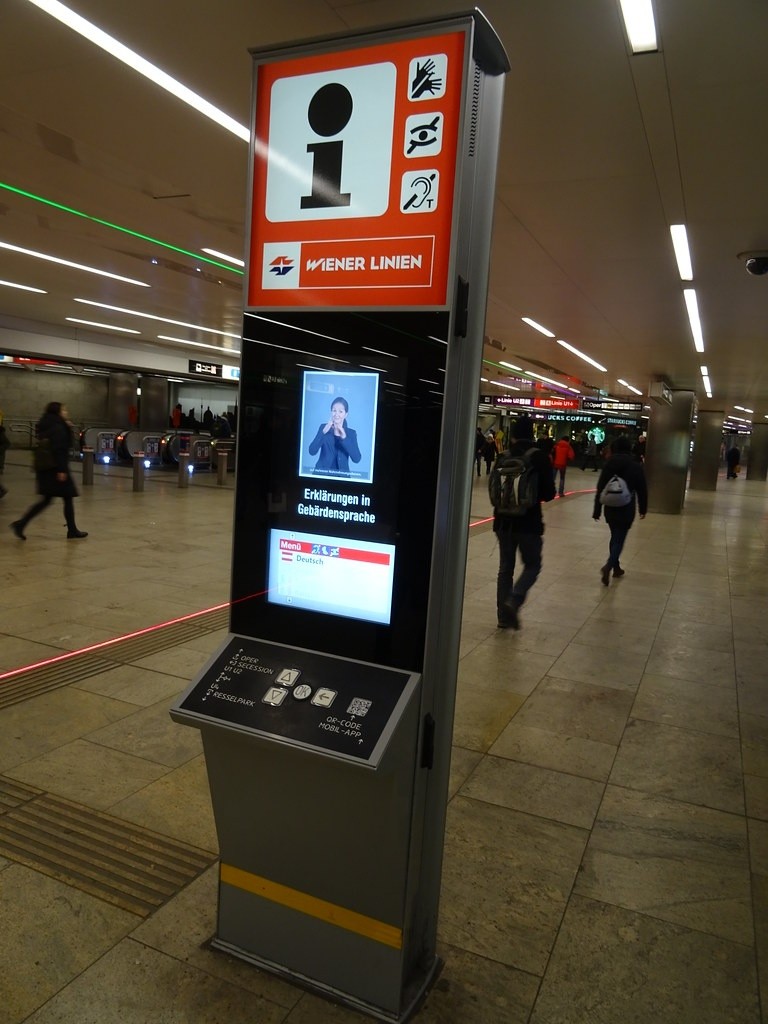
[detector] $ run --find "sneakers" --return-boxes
[498,599,520,629]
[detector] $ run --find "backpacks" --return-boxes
[487,447,542,517]
[599,457,636,507]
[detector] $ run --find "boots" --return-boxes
[601,561,611,585]
[613,562,626,579]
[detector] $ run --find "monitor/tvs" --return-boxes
[227,311,450,674]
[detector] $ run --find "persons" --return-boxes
[309,397,361,478]
[727,444,740,479]
[204,406,234,438]
[579,434,647,473]
[8,402,88,541]
[488,417,556,630]
[592,438,647,586]
[475,427,574,498]
[0,426,11,499]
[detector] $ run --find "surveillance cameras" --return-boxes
[746,258,768,276]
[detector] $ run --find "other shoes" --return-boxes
[559,494,565,497]
[8,519,27,539]
[67,529,88,539]
[580,466,585,470]
[592,469,598,472]
